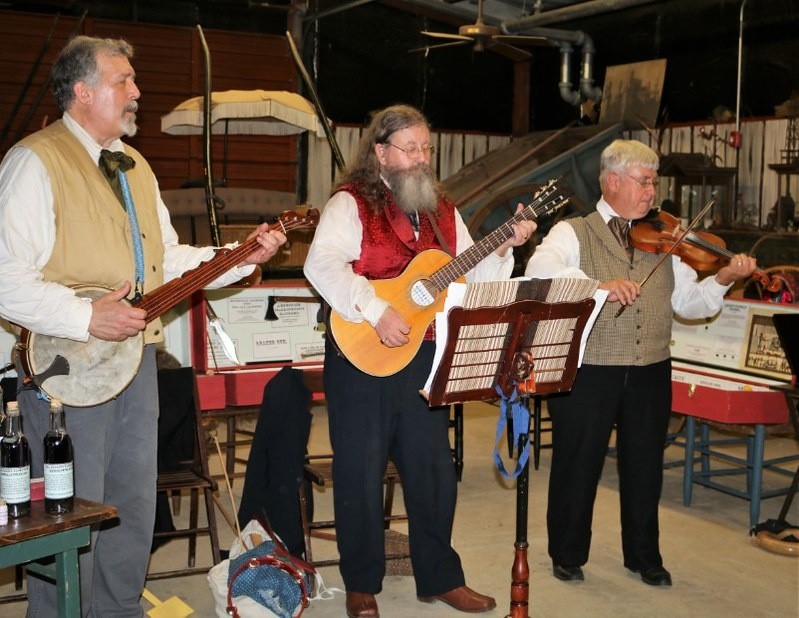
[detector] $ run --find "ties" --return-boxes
[407,210,418,232]
[607,216,633,267]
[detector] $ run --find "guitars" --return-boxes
[325,177,574,377]
[21,202,321,407]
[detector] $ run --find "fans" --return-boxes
[410,0,548,69]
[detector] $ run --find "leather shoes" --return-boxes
[552,561,585,584]
[623,558,673,588]
[417,586,496,613]
[345,591,380,618]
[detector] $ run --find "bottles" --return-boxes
[43,398,76,515]
[0,401,32,520]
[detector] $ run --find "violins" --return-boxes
[628,205,796,304]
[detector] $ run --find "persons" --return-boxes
[525,139,757,585]
[303,105,538,617]
[0,36,287,618]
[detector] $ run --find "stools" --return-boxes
[447,385,799,530]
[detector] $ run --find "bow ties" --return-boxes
[100,149,137,179]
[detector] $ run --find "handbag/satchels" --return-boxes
[207,518,334,618]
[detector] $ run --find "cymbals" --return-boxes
[225,556,310,618]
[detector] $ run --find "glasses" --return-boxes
[620,171,661,189]
[383,141,437,160]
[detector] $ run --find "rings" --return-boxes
[380,337,386,343]
[737,263,741,265]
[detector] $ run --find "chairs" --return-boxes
[0,367,413,598]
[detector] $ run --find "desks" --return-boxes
[0,496,119,618]
[674,167,738,225]
[767,163,799,231]
